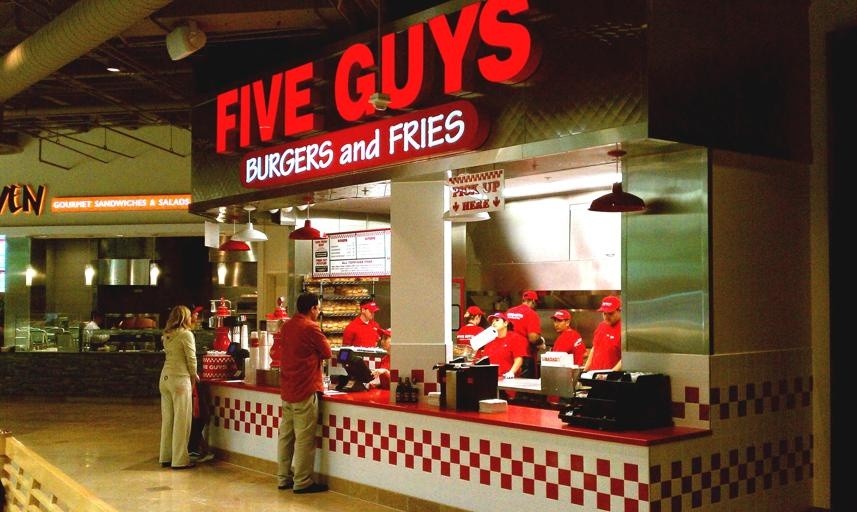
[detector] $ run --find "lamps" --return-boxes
[287,196,320,240]
[441,209,491,223]
[586,145,648,213]
[217,220,251,252]
[229,205,269,242]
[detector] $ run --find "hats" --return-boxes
[466,305,486,316]
[596,296,622,313]
[486,312,508,323]
[360,301,381,313]
[523,290,539,301]
[550,310,571,321]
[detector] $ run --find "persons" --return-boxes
[277,293,332,494]
[537,309,588,404]
[371,327,392,389]
[159,305,200,470]
[342,299,382,347]
[583,296,622,372]
[188,306,215,464]
[505,291,541,378]
[456,305,486,364]
[476,313,529,399]
[82,311,105,342]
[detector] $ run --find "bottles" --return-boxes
[315,244,328,273]
[396,377,418,403]
[82,328,90,350]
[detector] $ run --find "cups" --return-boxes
[228,315,248,350]
[245,331,274,385]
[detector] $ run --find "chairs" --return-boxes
[11,316,85,352]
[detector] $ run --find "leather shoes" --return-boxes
[278,483,295,490]
[292,483,328,494]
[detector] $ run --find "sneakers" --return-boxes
[159,461,172,467]
[171,462,197,469]
[187,449,201,459]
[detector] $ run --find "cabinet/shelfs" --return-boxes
[302,279,377,366]
